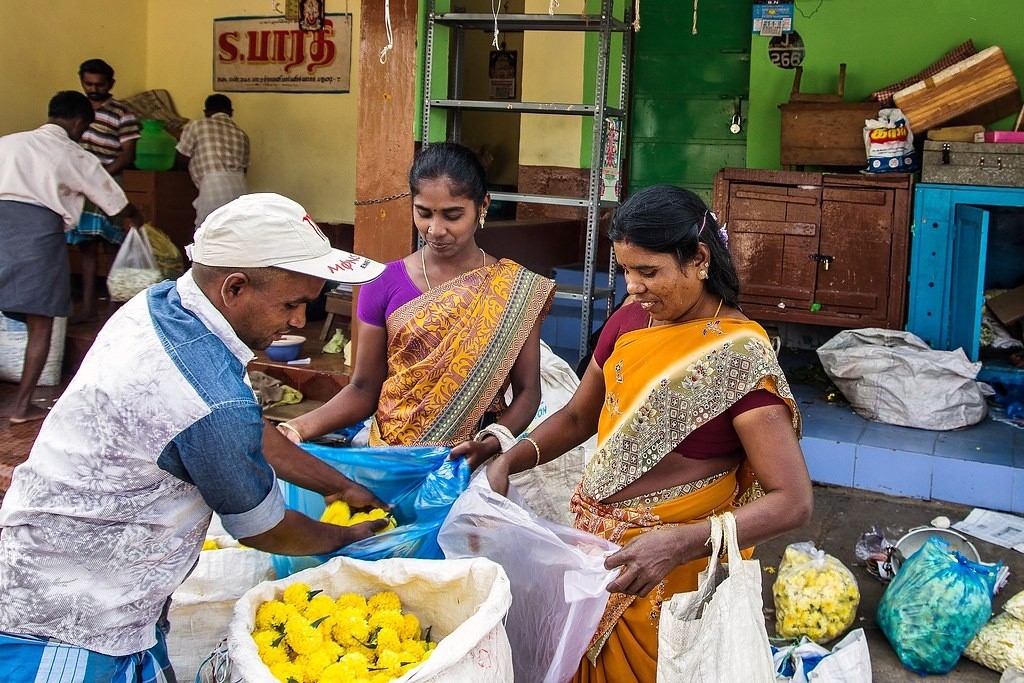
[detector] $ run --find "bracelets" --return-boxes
[279,423,303,442]
[719,515,727,558]
[521,438,540,466]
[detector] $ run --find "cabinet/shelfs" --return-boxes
[905,184,1024,386]
[707,167,919,329]
[408,0,635,369]
[66,169,193,278]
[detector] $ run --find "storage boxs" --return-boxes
[778,46,1024,189]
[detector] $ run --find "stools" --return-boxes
[318,288,358,338]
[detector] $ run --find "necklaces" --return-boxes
[648,298,723,328]
[422,246,486,289]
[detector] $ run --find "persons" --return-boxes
[66,59,140,322]
[0,192,390,683]
[0,91,142,426]
[468,185,813,683]
[278,142,557,472]
[172,94,250,227]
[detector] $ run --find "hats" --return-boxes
[184,192,386,285]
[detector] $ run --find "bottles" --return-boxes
[133,120,177,169]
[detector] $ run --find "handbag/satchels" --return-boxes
[858,107,922,174]
[772,541,861,643]
[106,226,163,302]
[653,511,778,683]
[771,627,873,683]
[877,535,1003,675]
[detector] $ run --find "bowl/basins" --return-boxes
[263,334,306,362]
[891,529,980,579]
